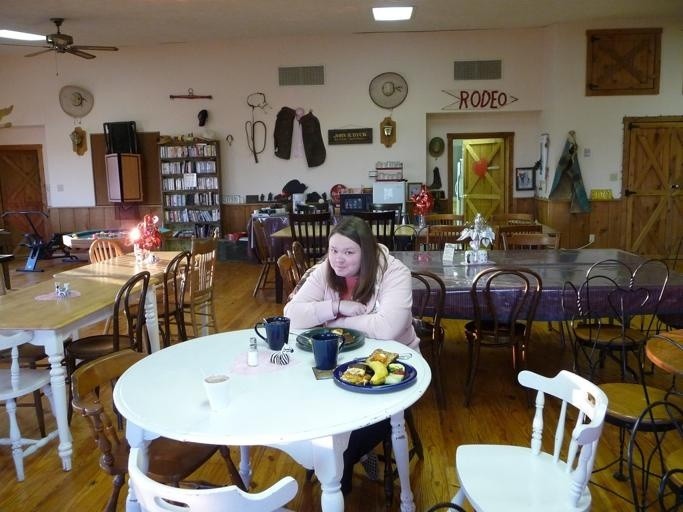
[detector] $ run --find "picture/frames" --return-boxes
[515,167,535,190]
[409,183,422,201]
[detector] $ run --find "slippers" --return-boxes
[362,449,383,482]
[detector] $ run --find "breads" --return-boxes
[367,348,399,367]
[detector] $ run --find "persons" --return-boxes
[282,214,420,496]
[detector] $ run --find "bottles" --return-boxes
[474,213,481,229]
[136,253,156,263]
[248,337,257,367]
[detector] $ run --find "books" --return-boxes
[160,142,220,238]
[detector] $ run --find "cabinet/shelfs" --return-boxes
[158,139,221,240]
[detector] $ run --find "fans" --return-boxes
[0,18,118,60]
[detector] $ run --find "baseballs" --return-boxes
[319,199,324,204]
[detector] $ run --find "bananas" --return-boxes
[366,356,388,385]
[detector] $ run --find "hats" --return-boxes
[369,71,409,109]
[59,85,94,118]
[429,136,445,158]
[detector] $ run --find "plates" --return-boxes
[333,357,417,392]
[296,327,364,349]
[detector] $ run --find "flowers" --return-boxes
[409,186,435,215]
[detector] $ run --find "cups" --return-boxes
[312,332,345,370]
[255,317,290,351]
[465,250,487,264]
[376,161,402,180]
[55,282,70,297]
[254,208,285,214]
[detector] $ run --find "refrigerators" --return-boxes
[373,181,407,224]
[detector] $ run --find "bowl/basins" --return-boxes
[558,250,580,263]
